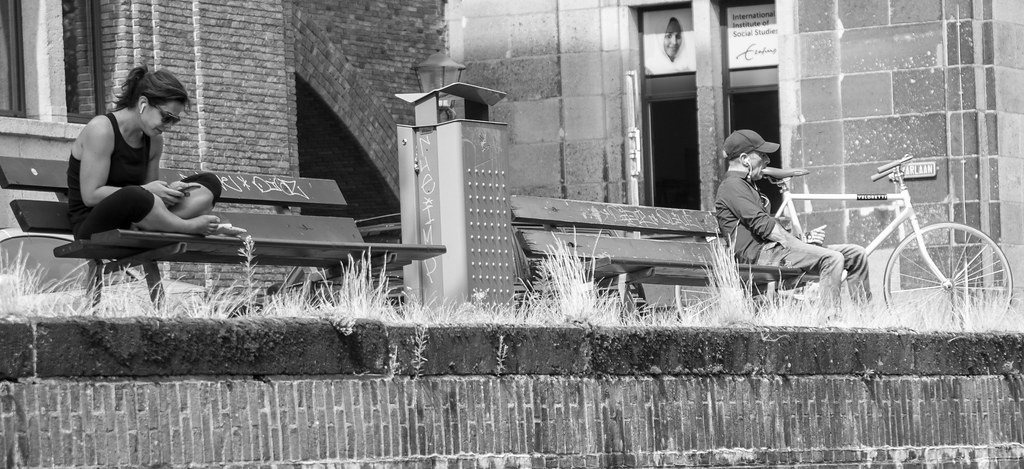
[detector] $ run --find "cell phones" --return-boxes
[177,185,201,192]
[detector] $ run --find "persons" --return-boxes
[716,129,872,321]
[67,66,223,240]
[648,14,689,74]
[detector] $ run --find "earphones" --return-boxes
[139,103,147,114]
[744,158,748,164]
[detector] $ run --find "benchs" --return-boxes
[0,156,448,316]
[510,195,804,315]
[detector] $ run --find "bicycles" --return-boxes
[754,156,1014,330]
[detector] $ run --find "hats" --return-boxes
[723,129,780,160]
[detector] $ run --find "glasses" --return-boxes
[154,104,181,125]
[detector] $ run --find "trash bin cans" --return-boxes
[393,80,516,308]
